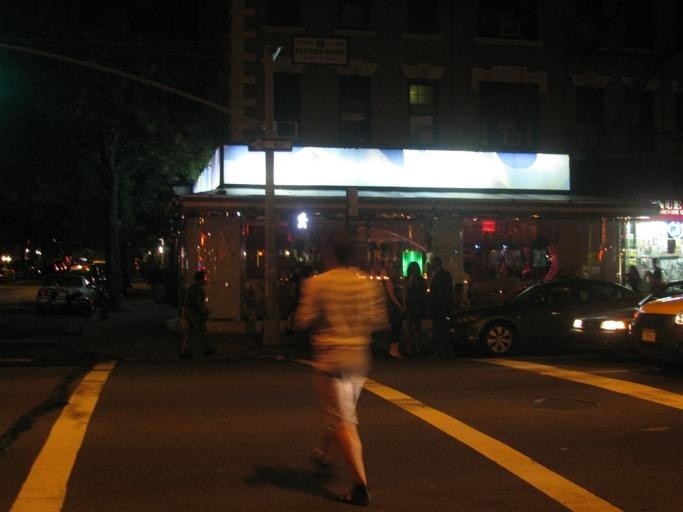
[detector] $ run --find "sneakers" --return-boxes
[311,446,372,507]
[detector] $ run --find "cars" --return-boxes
[37,272,99,318]
[444,279,683,369]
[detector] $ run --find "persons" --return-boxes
[624,266,641,290]
[179,270,212,359]
[269,251,525,365]
[644,257,661,293]
[294,228,389,506]
